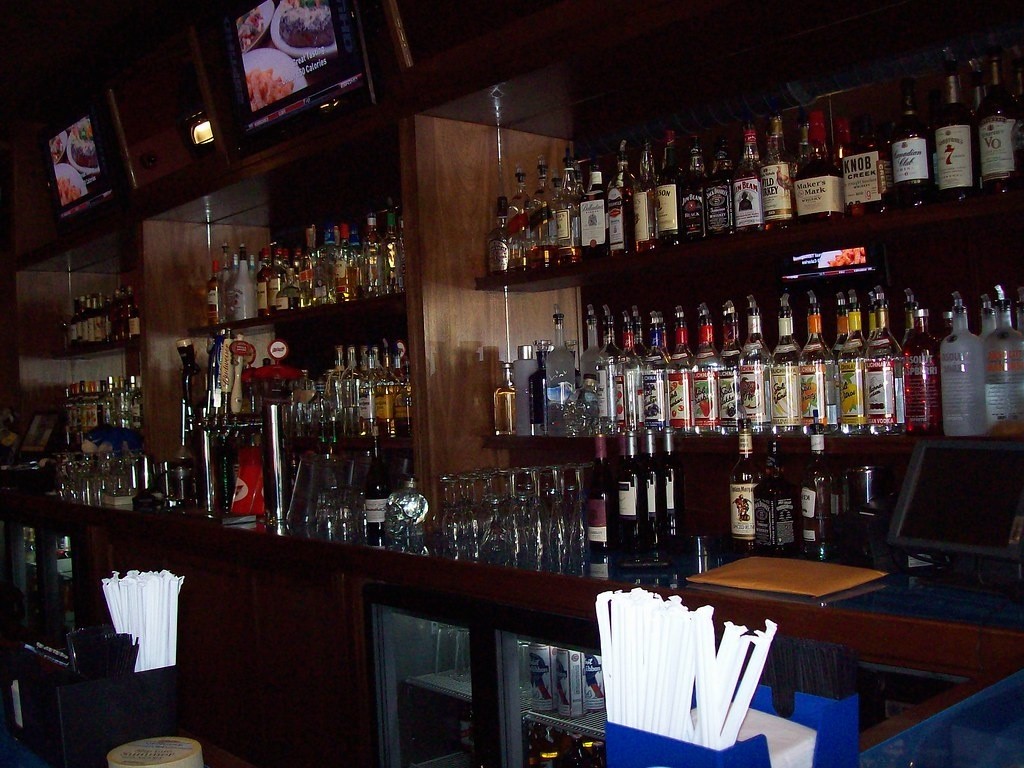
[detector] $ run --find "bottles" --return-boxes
[58,536,73,599]
[484,48,1024,273]
[65,283,142,354]
[523,721,606,768]
[0,520,5,582]
[493,285,1024,441]
[10,525,39,591]
[209,336,413,438]
[210,426,264,515]
[384,477,429,557]
[729,417,832,561]
[61,374,143,433]
[585,428,688,557]
[206,205,406,325]
[461,700,474,753]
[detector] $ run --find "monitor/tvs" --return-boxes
[215,0,377,146]
[41,105,121,227]
[886,440,1024,599]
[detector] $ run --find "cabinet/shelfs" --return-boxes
[11,219,142,515]
[411,0,1023,636]
[143,120,408,548]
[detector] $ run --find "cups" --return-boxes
[51,451,149,507]
[689,533,709,555]
[433,624,471,683]
[317,486,364,542]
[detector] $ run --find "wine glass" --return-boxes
[432,461,595,577]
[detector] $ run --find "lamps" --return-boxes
[185,108,214,148]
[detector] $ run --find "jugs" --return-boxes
[155,455,204,501]
[287,458,357,536]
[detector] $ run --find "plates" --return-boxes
[49,130,68,165]
[242,48,309,128]
[52,163,88,218]
[270,1,337,58]
[235,0,275,57]
[67,119,100,174]
